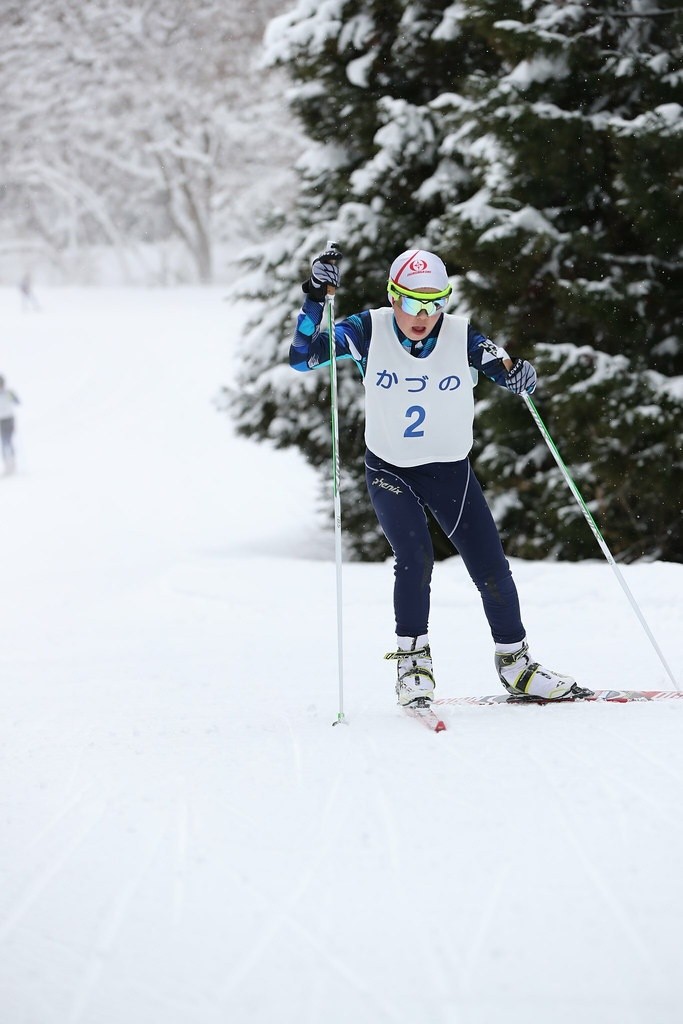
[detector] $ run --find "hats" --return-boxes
[388,250,448,305]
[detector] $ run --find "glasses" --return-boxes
[388,281,452,317]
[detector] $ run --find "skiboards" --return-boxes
[405,690,683,732]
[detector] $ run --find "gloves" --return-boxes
[505,360,537,395]
[302,251,343,302]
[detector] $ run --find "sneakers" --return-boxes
[396,632,433,707]
[496,636,576,699]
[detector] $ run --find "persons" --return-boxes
[0,375,20,477]
[289,249,576,707]
